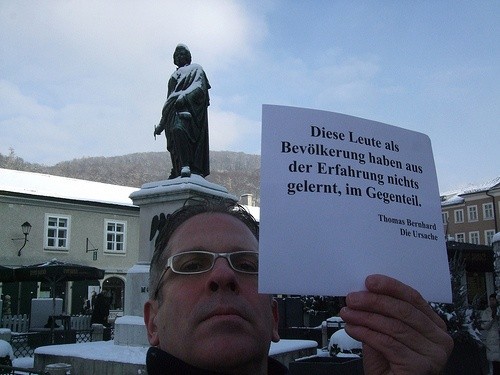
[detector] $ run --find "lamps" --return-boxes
[18,221,32,256]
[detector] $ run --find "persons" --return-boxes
[154,46,210,180]
[480,293,500,375]
[143,195,454,375]
[91,290,122,310]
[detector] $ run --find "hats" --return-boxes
[489,293,497,298]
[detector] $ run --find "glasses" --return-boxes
[152,251,259,299]
